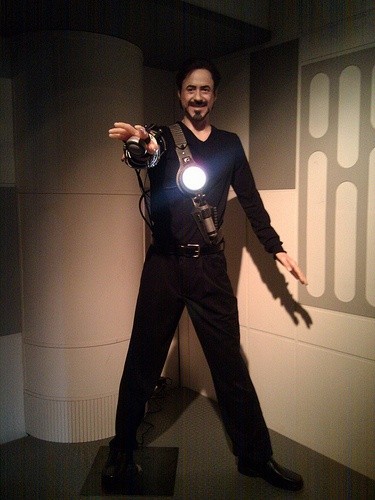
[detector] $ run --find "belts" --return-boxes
[151,239,225,258]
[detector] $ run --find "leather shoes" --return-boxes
[237,457,303,491]
[102,447,136,491]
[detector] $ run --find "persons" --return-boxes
[100,52,309,495]
